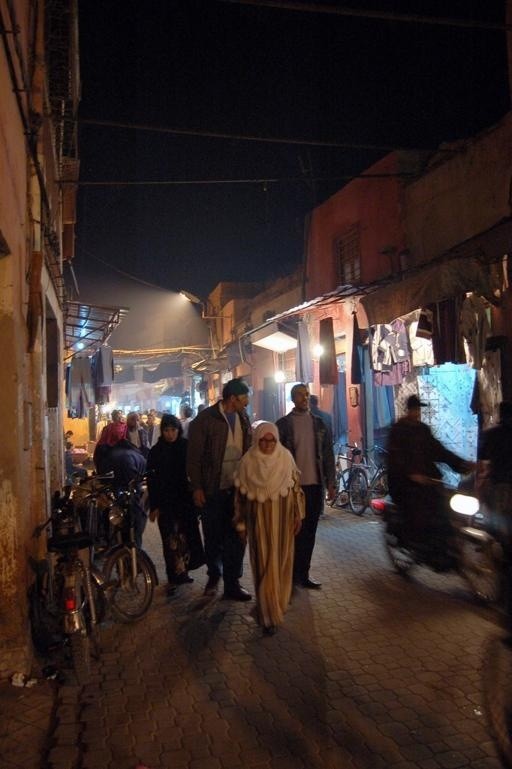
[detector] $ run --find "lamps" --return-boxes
[179,291,232,320]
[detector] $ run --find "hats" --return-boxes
[408,396,427,407]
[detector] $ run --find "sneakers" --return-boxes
[203,577,218,597]
[167,575,193,595]
[224,586,251,602]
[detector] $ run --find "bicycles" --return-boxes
[31,483,109,677]
[323,439,396,515]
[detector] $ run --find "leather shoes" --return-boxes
[301,576,323,589]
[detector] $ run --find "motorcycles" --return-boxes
[369,460,512,605]
[59,453,164,625]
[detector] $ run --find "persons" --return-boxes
[230,419,306,637]
[60,396,206,496]
[146,413,208,584]
[88,439,148,576]
[275,384,334,588]
[187,378,252,600]
[309,393,332,516]
[477,400,511,565]
[385,395,489,572]
